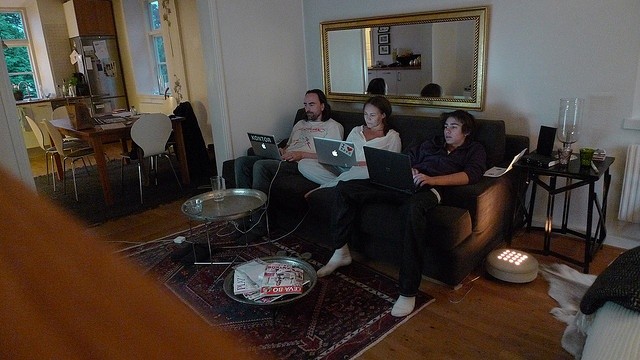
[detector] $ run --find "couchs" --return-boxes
[222,107,529,290]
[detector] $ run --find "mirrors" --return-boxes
[319,5,489,111]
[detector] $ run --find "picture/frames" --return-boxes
[378,33,391,44]
[378,44,391,55]
[378,26,390,33]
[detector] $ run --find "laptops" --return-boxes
[312,137,357,169]
[363,147,423,195]
[247,130,292,161]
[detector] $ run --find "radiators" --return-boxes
[617,143,640,222]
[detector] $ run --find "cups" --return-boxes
[210,176,226,202]
[185,199,204,216]
[558,146,572,166]
[579,146,595,167]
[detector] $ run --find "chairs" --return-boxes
[42,118,112,201]
[119,113,184,204]
[25,116,96,191]
[51,105,94,121]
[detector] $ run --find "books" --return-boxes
[232,256,311,303]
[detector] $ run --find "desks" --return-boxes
[15,94,91,105]
[512,149,616,277]
[47,109,191,202]
[181,188,271,271]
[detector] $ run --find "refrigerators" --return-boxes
[68,35,130,118]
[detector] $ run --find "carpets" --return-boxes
[35,153,217,226]
[111,221,435,358]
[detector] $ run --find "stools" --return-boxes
[486,248,539,285]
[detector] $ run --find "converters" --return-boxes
[173,236,185,244]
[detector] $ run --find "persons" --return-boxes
[483,147,528,180]
[317,109,486,318]
[230,89,345,247]
[298,95,401,198]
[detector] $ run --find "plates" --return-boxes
[180,188,268,223]
[222,256,319,307]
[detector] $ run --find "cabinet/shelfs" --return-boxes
[368,65,421,71]
[16,102,57,148]
